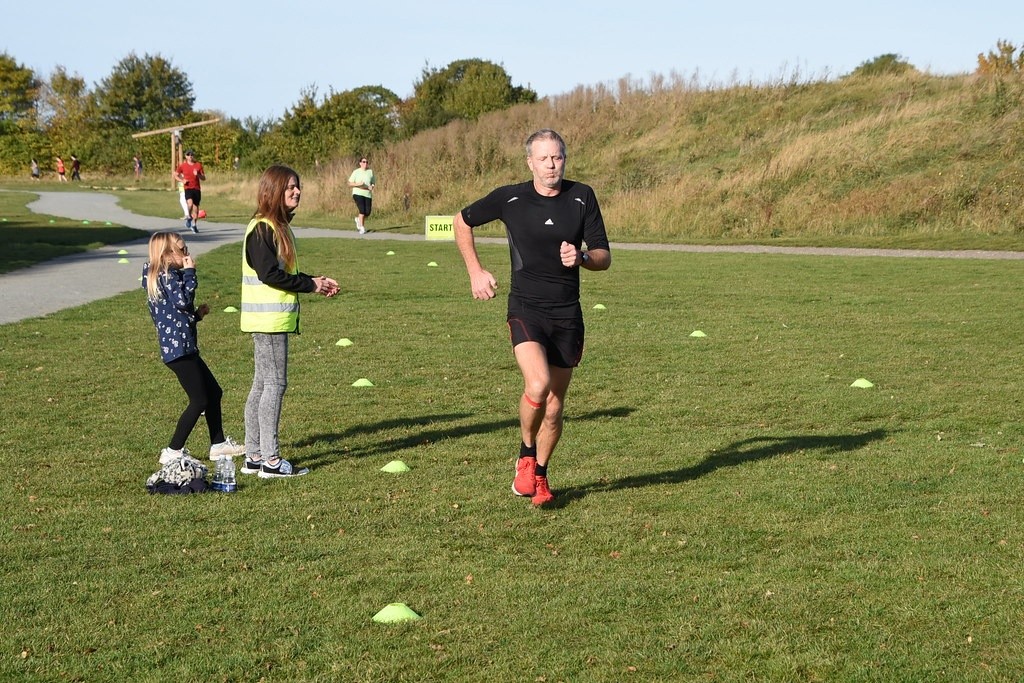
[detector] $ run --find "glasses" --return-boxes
[186,153,193,156]
[363,161,368,163]
[170,246,188,256]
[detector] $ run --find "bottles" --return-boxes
[223,456,236,493]
[213,456,223,490]
[219,455,226,479]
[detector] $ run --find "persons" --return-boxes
[133,156,142,179]
[239,166,341,477]
[69,155,83,182]
[348,157,375,234]
[141,231,245,463]
[55,156,68,182]
[174,151,206,234]
[454,129,612,506]
[178,174,190,220]
[30,158,40,181]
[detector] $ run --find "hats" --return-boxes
[186,150,193,155]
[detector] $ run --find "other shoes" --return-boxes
[359,226,366,234]
[355,216,361,230]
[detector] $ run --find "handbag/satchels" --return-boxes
[146,456,207,495]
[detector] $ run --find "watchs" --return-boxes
[580,251,589,265]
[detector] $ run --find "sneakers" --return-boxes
[241,457,263,475]
[191,226,198,234]
[209,436,246,461]
[533,476,554,509]
[186,216,191,228]
[158,448,202,465]
[511,456,538,497]
[258,458,309,479]
